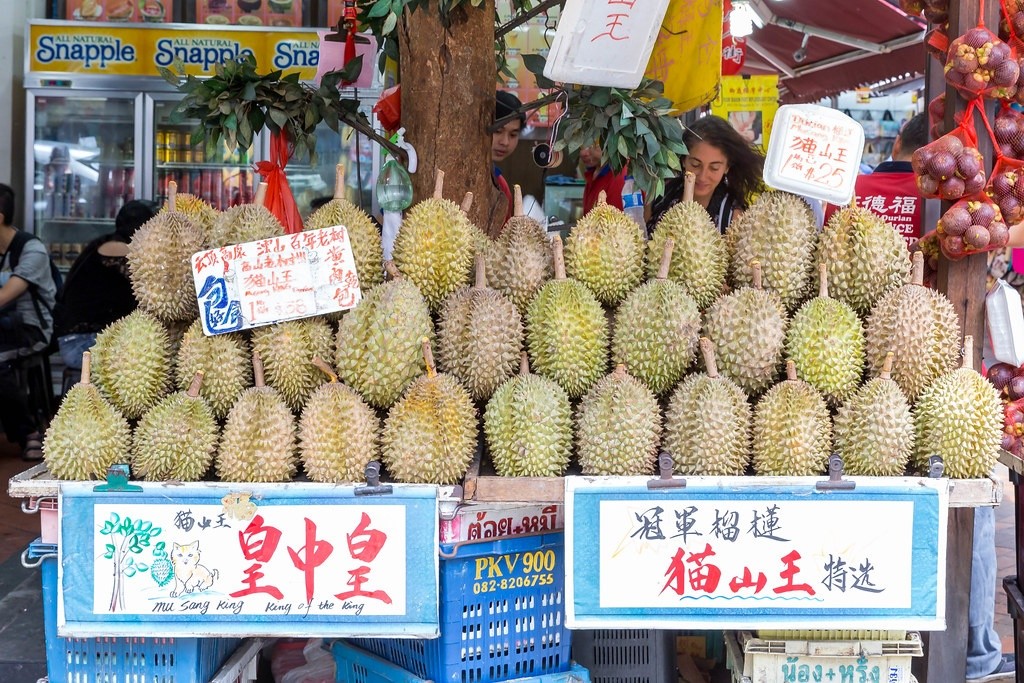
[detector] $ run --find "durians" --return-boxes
[41,164,1006,488]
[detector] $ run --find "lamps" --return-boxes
[729,0,773,38]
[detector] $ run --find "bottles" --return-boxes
[621,175,648,243]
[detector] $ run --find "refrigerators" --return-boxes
[23,17,381,365]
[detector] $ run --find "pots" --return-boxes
[138,0,165,21]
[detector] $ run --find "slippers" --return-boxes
[21,436,44,462]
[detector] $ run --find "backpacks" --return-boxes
[6,232,65,331]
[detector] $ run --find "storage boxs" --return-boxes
[322,637,590,683]
[36,638,268,683]
[571,630,671,683]
[339,528,570,683]
[676,636,706,659]
[22,540,242,683]
[67,0,172,24]
[725,631,924,683]
[196,0,302,31]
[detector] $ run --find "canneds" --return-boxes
[43,131,251,266]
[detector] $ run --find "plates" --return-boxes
[239,15,262,26]
[106,8,135,22]
[206,15,229,25]
[74,5,102,21]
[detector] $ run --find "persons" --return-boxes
[490,90,528,224]
[648,114,766,235]
[984,220,1024,292]
[56,199,164,369]
[825,111,1017,683]
[0,184,58,459]
[580,140,645,218]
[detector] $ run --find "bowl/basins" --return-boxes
[269,0,292,14]
[238,0,262,12]
